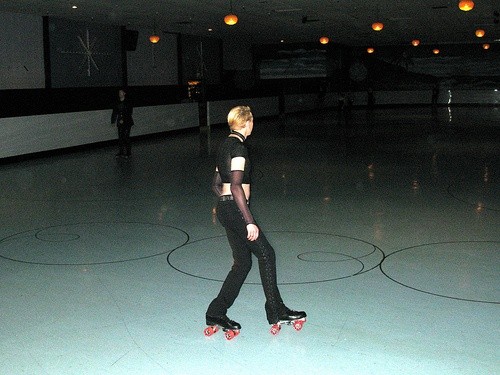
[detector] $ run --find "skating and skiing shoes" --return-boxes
[205,312,241,340]
[269,306,306,335]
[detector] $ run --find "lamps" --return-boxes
[149,16,160,43]
[475,26,485,38]
[372,5,384,31]
[459,0,474,11]
[433,41,440,55]
[319,24,329,45]
[366,37,375,53]
[483,43,490,49]
[224,0,239,25]
[412,30,420,46]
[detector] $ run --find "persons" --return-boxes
[204,105,307,340]
[110,90,134,162]
